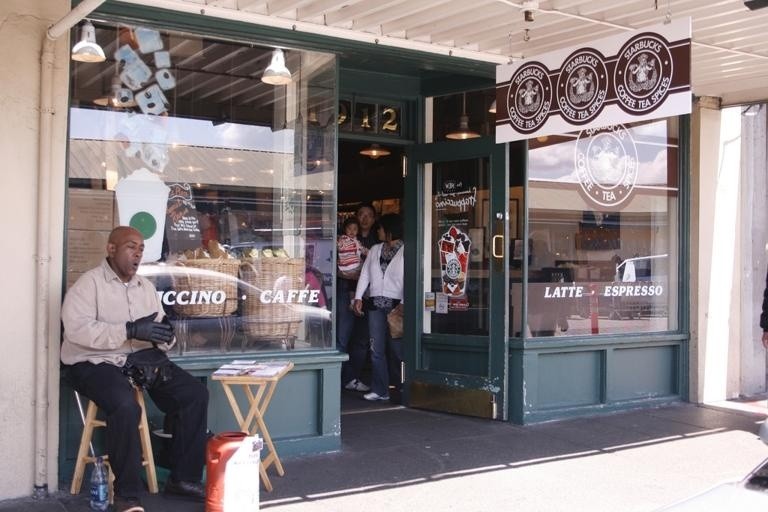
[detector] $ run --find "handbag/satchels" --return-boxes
[127,356,171,391]
[387,308,403,339]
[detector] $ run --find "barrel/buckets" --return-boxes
[205,431,264,512]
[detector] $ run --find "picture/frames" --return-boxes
[482,198,519,261]
[467,227,485,264]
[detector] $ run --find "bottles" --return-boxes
[90,456,109,510]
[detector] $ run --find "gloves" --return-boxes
[126,312,176,345]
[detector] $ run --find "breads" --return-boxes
[160,238,231,266]
[241,246,291,259]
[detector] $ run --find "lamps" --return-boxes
[70,19,109,67]
[259,46,295,89]
[93,22,141,111]
[357,142,393,161]
[443,93,483,143]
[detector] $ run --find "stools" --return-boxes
[212,363,294,493]
[70,385,160,496]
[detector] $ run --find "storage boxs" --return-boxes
[66,188,120,298]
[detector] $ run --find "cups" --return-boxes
[440,248,470,297]
[111,185,172,263]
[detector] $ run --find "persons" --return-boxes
[757,263,768,352]
[351,212,406,403]
[273,232,329,349]
[335,200,381,394]
[59,225,210,511]
[338,214,371,315]
[528,228,571,339]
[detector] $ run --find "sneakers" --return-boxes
[114,497,144,512]
[165,477,206,501]
[345,379,390,401]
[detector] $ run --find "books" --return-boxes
[213,357,292,378]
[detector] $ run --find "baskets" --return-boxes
[166,259,242,318]
[240,257,307,341]
